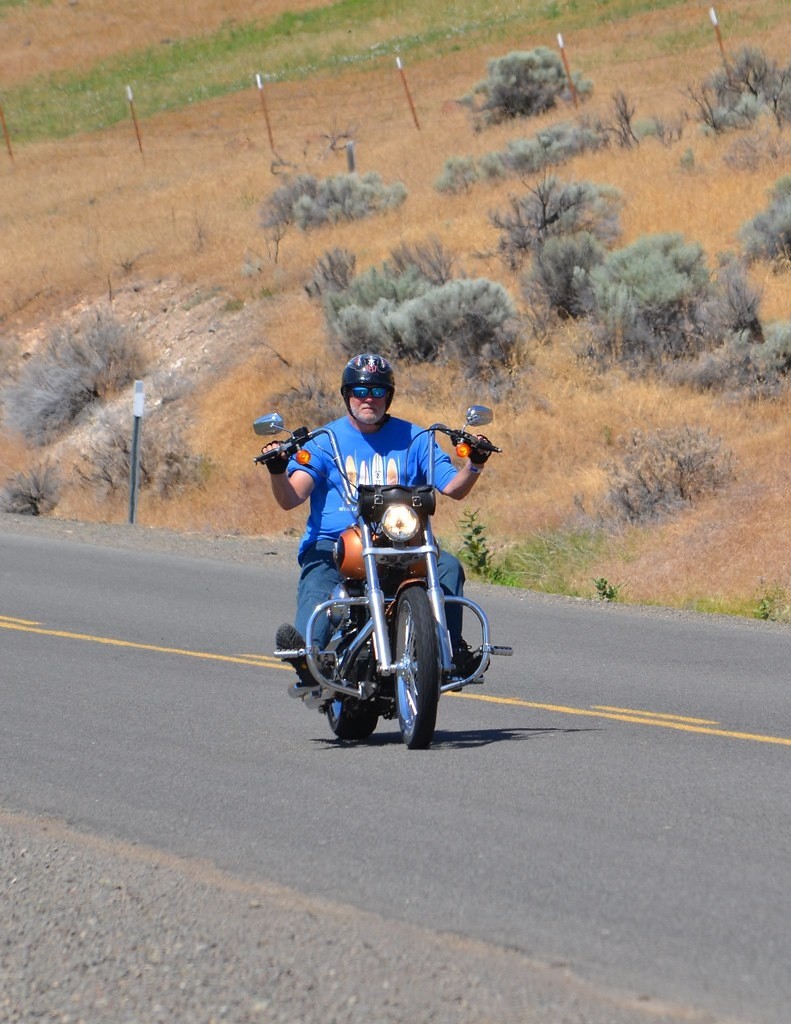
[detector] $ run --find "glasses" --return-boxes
[344,387,387,398]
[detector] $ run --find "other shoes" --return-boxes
[275,624,319,686]
[454,645,490,674]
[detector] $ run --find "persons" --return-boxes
[261,355,492,686]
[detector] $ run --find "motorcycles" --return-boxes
[253,403,513,751]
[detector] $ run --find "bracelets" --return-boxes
[467,462,484,473]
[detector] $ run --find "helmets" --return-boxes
[341,353,395,395]
